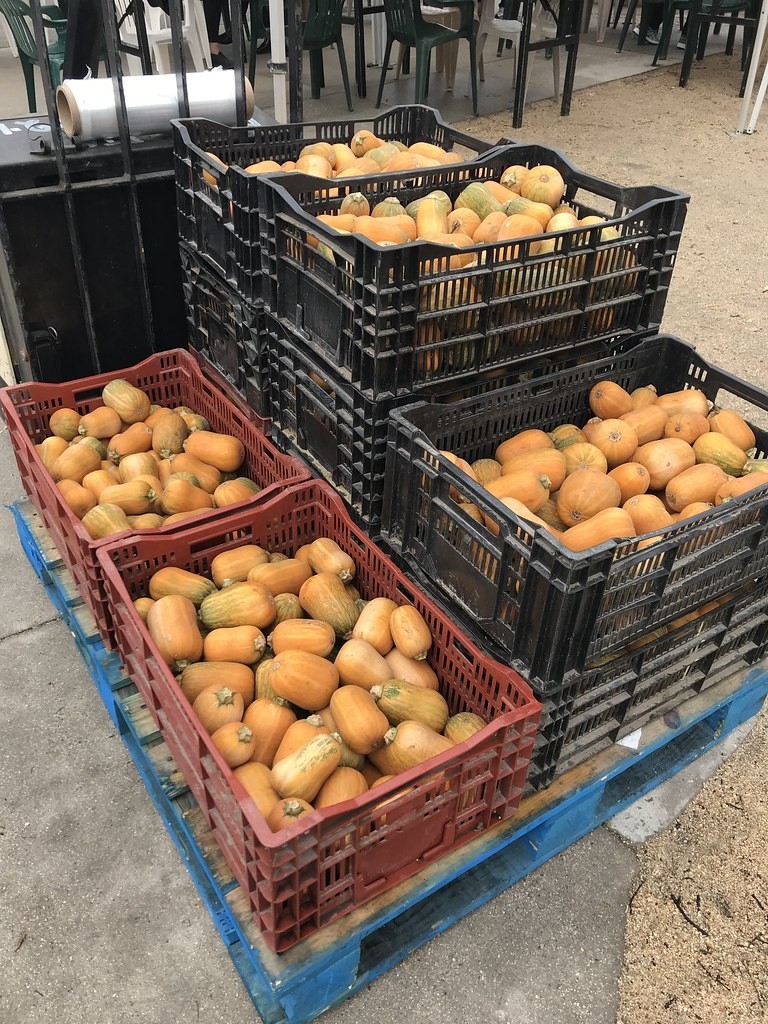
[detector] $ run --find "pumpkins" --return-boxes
[133,540,488,846]
[417,381,768,672]
[195,130,640,394]
[32,379,261,541]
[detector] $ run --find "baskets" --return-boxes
[389,333,767,801]
[96,479,543,953]
[1,349,311,651]
[170,104,689,551]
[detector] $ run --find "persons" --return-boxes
[252,7,290,56]
[632,0,699,51]
[149,0,234,69]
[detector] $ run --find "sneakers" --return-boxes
[633,23,660,44]
[677,36,698,50]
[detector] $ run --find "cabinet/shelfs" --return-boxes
[0,104,291,437]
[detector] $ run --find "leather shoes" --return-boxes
[211,51,234,68]
[256,38,271,54]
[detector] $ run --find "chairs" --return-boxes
[0,0,762,129]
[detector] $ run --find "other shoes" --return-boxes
[495,7,505,19]
[218,28,232,44]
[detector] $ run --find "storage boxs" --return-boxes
[0,102,768,955]
